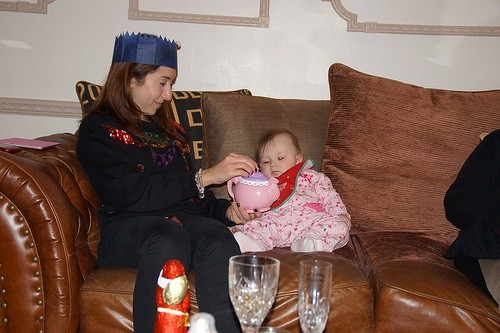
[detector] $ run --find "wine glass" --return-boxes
[227,255,281,333]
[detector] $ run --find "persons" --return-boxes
[76,33,262,333]
[444,128,500,310]
[234,130,351,253]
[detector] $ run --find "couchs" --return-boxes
[0,61,500,332]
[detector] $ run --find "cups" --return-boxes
[298,260,332,333]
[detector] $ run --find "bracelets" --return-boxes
[195,168,205,199]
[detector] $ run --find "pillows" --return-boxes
[318,63,499,236]
[76,80,252,171]
[198,91,330,201]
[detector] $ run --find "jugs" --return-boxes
[225,166,281,214]
